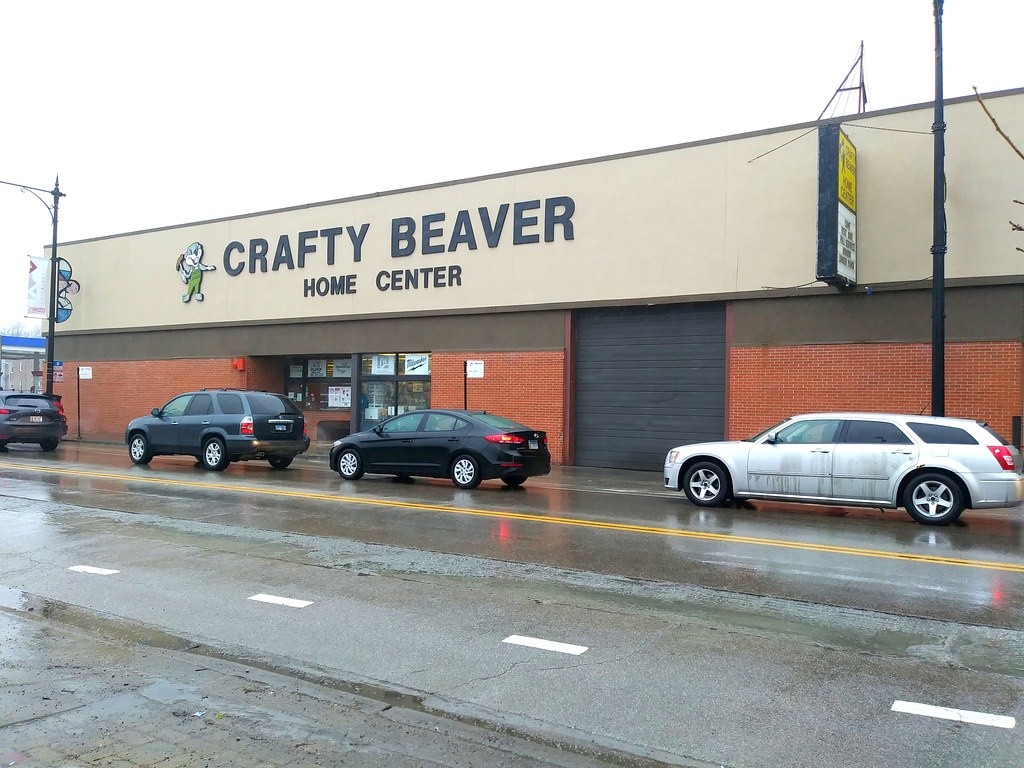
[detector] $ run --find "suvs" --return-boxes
[663,410,1024,526]
[0,391,63,452]
[125,387,310,472]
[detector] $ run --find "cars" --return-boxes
[330,409,552,489]
